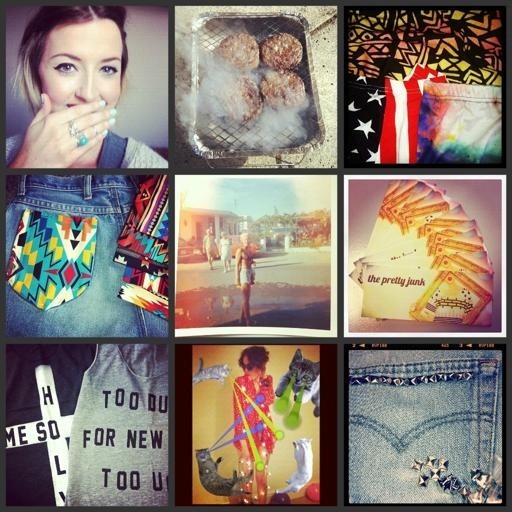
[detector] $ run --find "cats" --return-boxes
[193,357,232,385]
[275,348,321,417]
[195,447,254,496]
[275,438,313,494]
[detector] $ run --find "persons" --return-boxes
[234,230,259,328]
[203,228,220,270]
[232,346,276,504]
[3,5,172,171]
[219,231,233,275]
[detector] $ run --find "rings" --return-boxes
[76,131,92,151]
[67,120,79,133]
